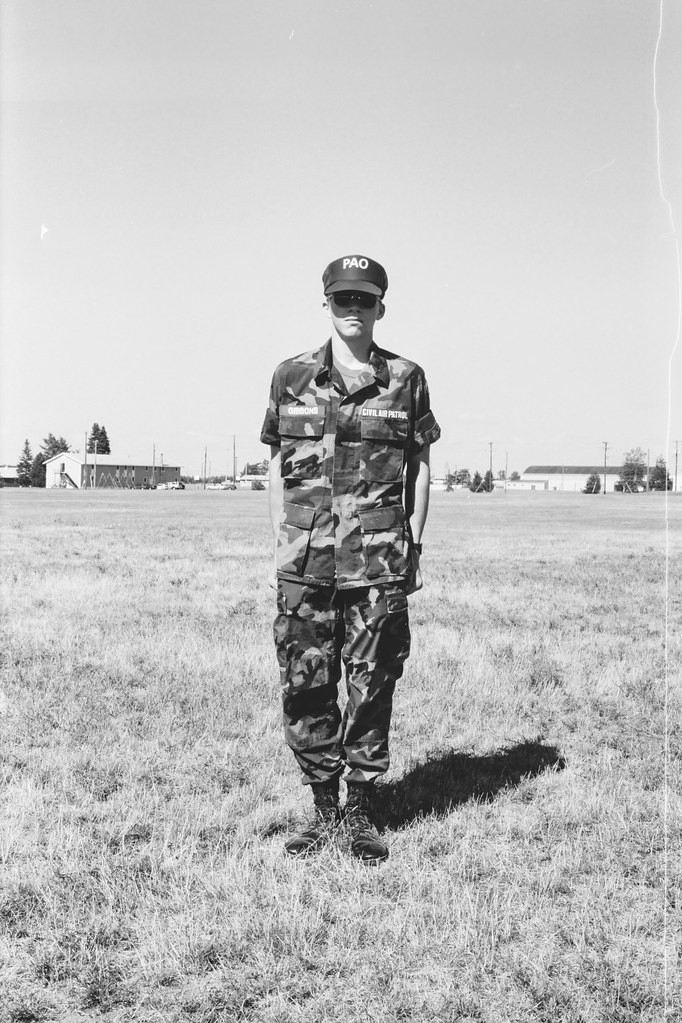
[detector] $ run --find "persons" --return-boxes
[267,252,430,863]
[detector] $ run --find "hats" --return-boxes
[323,255,388,299]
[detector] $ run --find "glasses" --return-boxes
[327,289,381,308]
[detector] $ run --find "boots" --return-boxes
[342,781,388,866]
[285,777,342,858]
